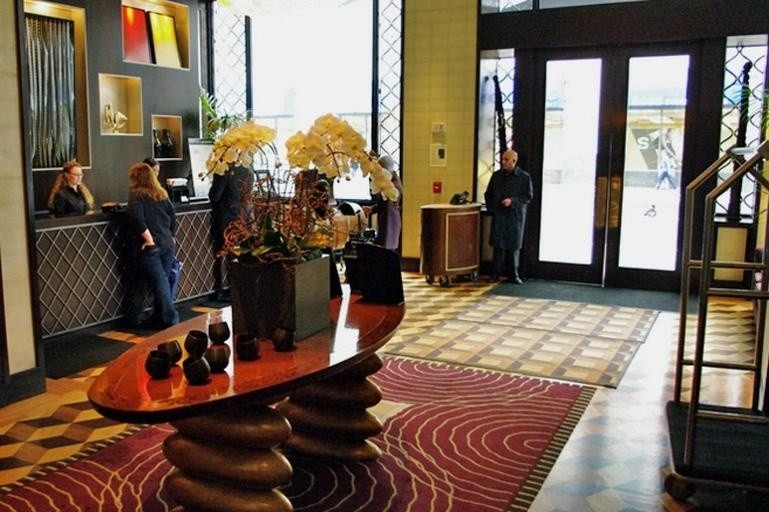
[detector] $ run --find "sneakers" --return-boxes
[487,274,499,283]
[509,277,522,284]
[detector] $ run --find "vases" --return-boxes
[228,252,332,342]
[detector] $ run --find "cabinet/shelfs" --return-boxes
[150,113,184,163]
[98,72,144,135]
[120,0,190,71]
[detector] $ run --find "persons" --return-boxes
[141,157,160,177]
[484,150,534,285]
[208,163,254,304]
[47,160,95,214]
[362,155,404,250]
[128,162,179,329]
[656,159,673,189]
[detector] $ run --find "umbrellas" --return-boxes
[168,238,185,300]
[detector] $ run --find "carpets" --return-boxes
[1,268,660,512]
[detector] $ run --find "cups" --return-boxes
[271,325,295,351]
[144,322,230,383]
[235,333,259,357]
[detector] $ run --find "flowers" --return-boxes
[198,112,403,268]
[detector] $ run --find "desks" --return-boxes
[86,281,408,512]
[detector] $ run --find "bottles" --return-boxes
[154,128,162,158]
[162,128,175,156]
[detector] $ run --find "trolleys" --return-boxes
[656,138,768,506]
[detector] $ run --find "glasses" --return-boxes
[70,172,84,177]
